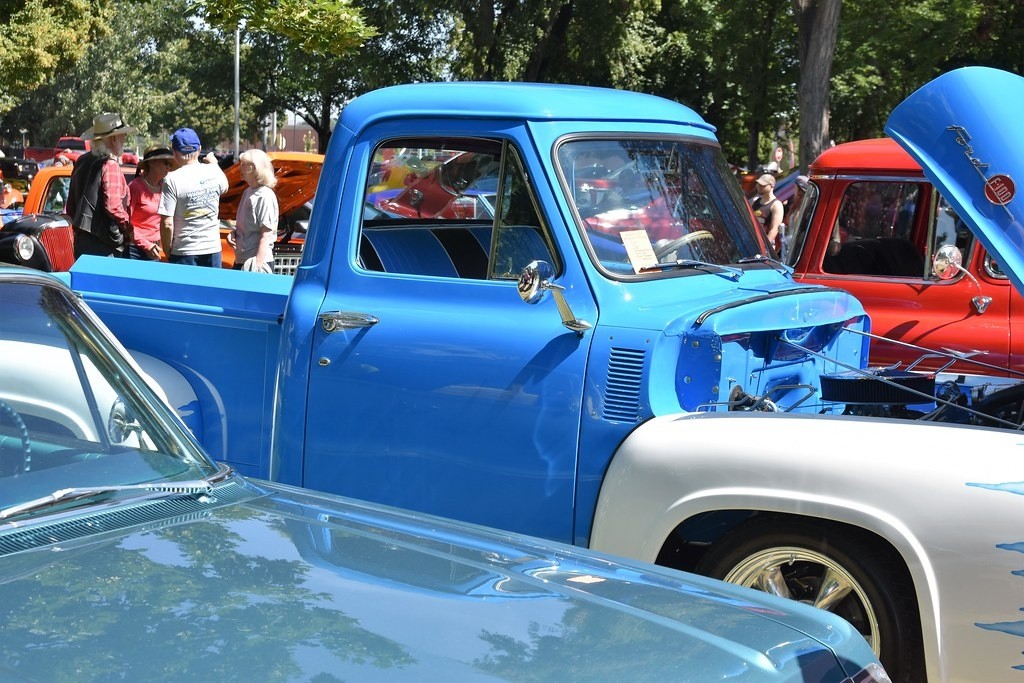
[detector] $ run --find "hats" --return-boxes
[134,144,178,178]
[794,176,812,192]
[170,128,200,153]
[754,174,775,188]
[80,112,137,140]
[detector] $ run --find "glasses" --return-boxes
[164,159,173,169]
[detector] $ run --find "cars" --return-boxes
[0,259,897,683]
[0,62,1024,682]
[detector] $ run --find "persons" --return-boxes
[782,176,809,264]
[227,148,279,274]
[48,156,70,211]
[157,128,229,268]
[64,113,138,259]
[127,144,180,262]
[751,174,783,251]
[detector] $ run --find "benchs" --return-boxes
[835,236,927,278]
[358,221,557,279]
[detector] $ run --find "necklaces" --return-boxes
[142,178,160,189]
